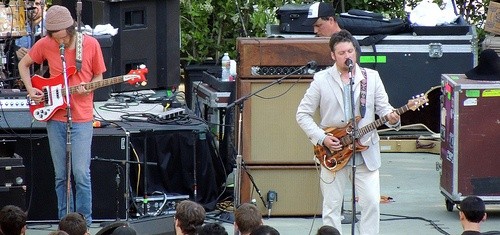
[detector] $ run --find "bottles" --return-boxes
[221,53,230,81]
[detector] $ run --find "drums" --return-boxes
[0,0,27,39]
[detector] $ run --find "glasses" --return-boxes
[172,213,176,220]
[78,212,86,222]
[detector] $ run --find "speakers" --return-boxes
[240,167,321,216]
[0,137,130,222]
[238,78,322,165]
[51,0,180,101]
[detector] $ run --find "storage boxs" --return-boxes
[439,74,500,211]
[192,81,231,108]
[267,25,478,139]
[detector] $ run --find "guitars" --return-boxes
[27,64,148,122]
[313,93,429,172]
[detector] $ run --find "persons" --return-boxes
[457,197,487,235]
[0,196,341,235]
[303,1,342,37]
[11,0,50,92]
[18,5,106,235]
[297,29,402,235]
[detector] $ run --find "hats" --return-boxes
[301,1,334,26]
[45,5,74,31]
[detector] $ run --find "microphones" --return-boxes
[345,59,352,68]
[59,44,65,58]
[266,191,277,217]
[306,61,318,68]
